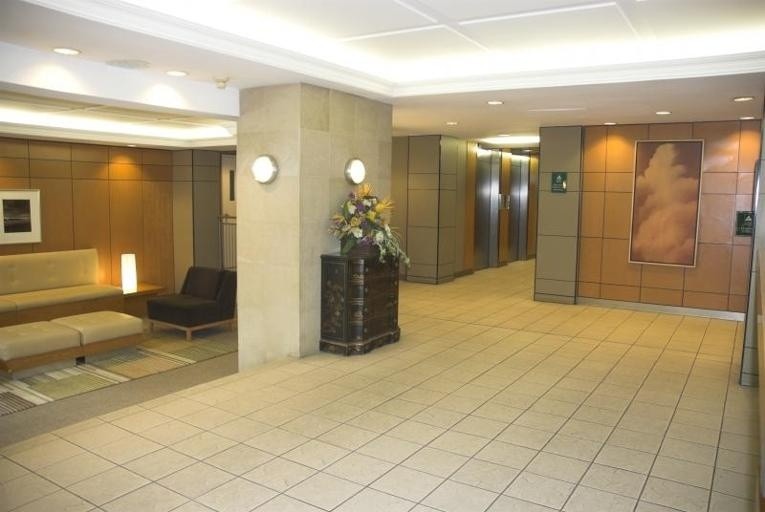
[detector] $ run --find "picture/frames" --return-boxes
[0,187,44,246]
[627,138,705,272]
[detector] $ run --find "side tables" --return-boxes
[119,281,164,319]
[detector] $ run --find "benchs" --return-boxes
[0,311,144,378]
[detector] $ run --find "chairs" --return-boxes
[147,264,237,342]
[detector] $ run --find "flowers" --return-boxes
[326,181,413,269]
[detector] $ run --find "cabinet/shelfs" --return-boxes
[319,252,402,357]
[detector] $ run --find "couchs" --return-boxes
[1,246,125,331]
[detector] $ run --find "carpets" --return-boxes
[0,328,238,418]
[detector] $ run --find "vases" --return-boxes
[341,236,380,257]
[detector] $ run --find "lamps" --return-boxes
[250,154,279,185]
[345,158,366,185]
[119,252,138,295]
